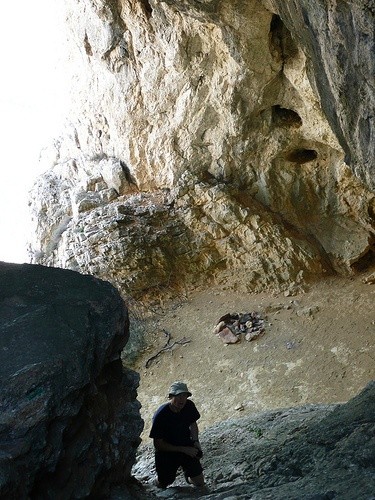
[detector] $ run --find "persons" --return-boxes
[148,382,206,487]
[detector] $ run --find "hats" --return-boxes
[167,382,192,398]
